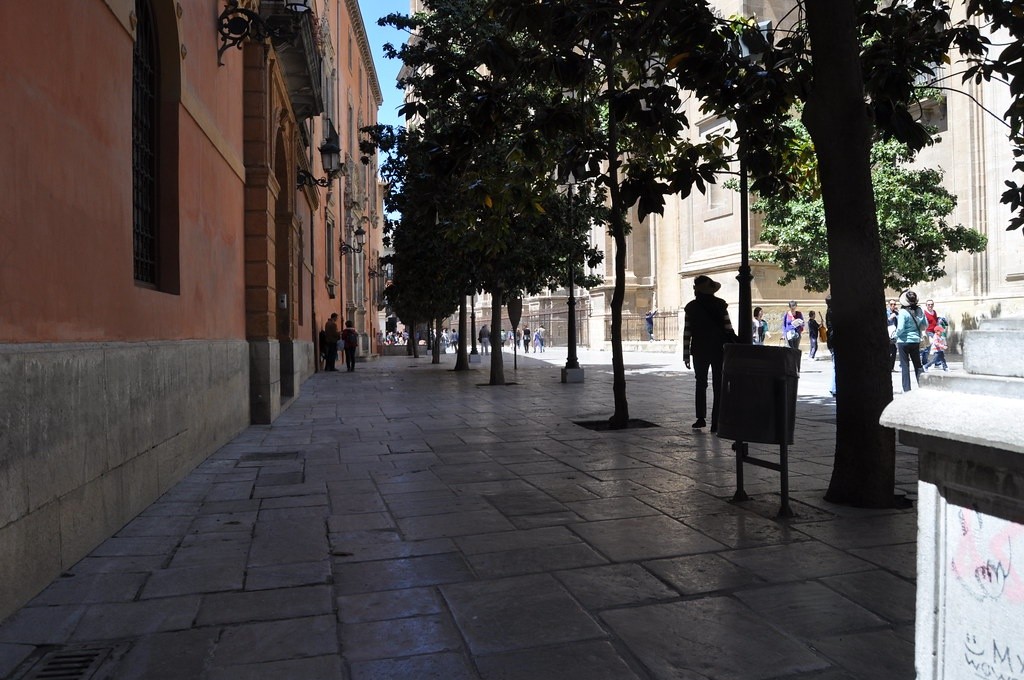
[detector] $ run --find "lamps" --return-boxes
[296,138,341,192]
[217,0,312,68]
[339,223,366,257]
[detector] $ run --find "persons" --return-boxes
[808,311,822,358]
[683,276,736,432]
[387,330,420,345]
[645,308,658,340]
[479,324,546,355]
[430,328,459,354]
[325,313,359,371]
[781,301,805,378]
[825,294,837,398]
[752,307,771,345]
[886,291,952,392]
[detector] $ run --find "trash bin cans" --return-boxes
[717,343,801,445]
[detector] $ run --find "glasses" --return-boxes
[890,304,896,306]
[928,304,933,306]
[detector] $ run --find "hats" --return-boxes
[693,276,722,296]
[934,325,944,332]
[900,292,919,307]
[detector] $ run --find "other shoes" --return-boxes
[351,366,354,372]
[328,368,339,371]
[934,362,941,368]
[924,366,928,373]
[325,369,329,371]
[348,367,351,372]
[692,420,707,428]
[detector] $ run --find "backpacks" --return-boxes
[319,330,327,360]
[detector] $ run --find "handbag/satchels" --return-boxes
[786,330,799,341]
[337,340,344,351]
[919,331,926,352]
[819,326,827,343]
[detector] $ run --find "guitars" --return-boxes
[818,311,827,343]
